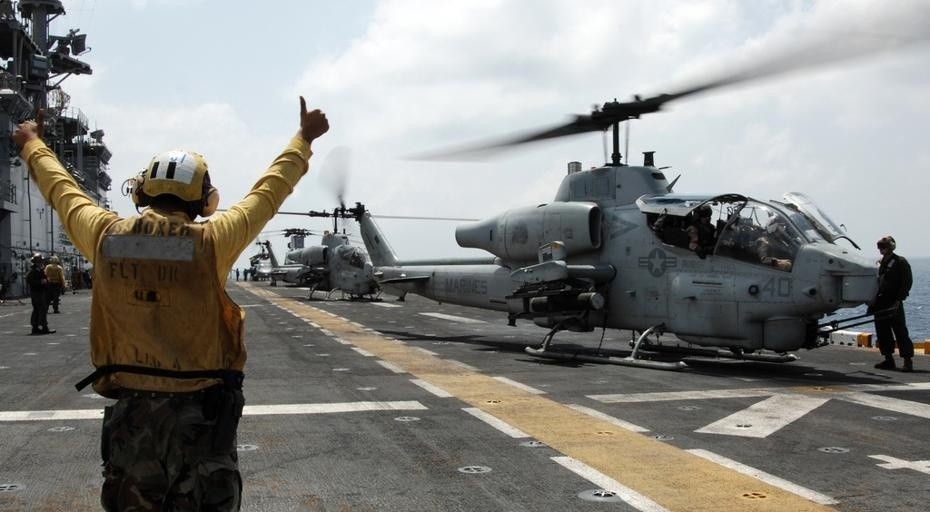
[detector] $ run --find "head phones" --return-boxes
[884,237,895,250]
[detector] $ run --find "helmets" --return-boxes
[878,236,896,250]
[49,254,60,262]
[767,214,786,234]
[693,204,712,215]
[32,253,44,262]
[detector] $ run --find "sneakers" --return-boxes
[32,327,56,335]
[902,359,912,371]
[876,359,895,368]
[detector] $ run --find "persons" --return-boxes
[243,267,249,282]
[235,268,240,282]
[687,204,720,260]
[12,96,330,510]
[25,253,56,335]
[867,235,915,372]
[44,256,66,314]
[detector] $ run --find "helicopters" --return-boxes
[206,203,484,305]
[255,225,366,295]
[318,1,930,376]
[243,239,283,282]
